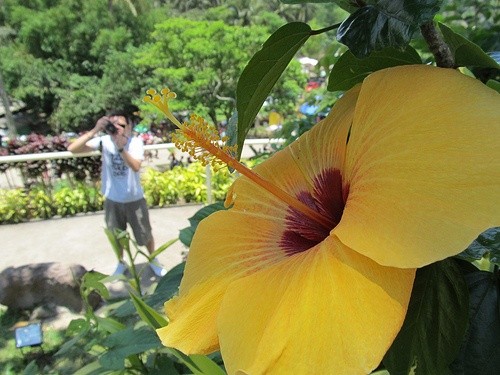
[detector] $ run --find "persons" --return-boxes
[67,111,168,278]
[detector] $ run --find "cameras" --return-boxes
[105,124,116,134]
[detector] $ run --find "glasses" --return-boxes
[120,124,128,128]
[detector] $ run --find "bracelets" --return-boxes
[118,148,123,152]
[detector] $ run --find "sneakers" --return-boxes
[110,262,129,283]
[148,258,167,277]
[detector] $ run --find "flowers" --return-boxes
[142,64,500,375]
